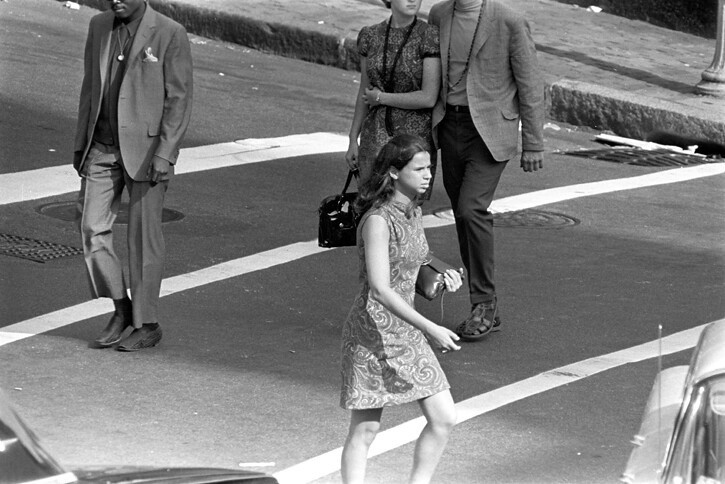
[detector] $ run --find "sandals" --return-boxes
[457,298,501,338]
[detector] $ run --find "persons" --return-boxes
[344,0,442,207]
[340,133,464,484]
[428,0,544,338]
[74,0,194,352]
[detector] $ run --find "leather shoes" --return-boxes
[115,323,163,351]
[95,305,133,347]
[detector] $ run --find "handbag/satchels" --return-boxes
[414,253,465,301]
[319,163,366,247]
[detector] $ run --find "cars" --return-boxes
[619,317,725,484]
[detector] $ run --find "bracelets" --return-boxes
[375,92,381,104]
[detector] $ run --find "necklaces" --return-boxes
[116,30,130,61]
[447,2,484,88]
[383,15,418,137]
